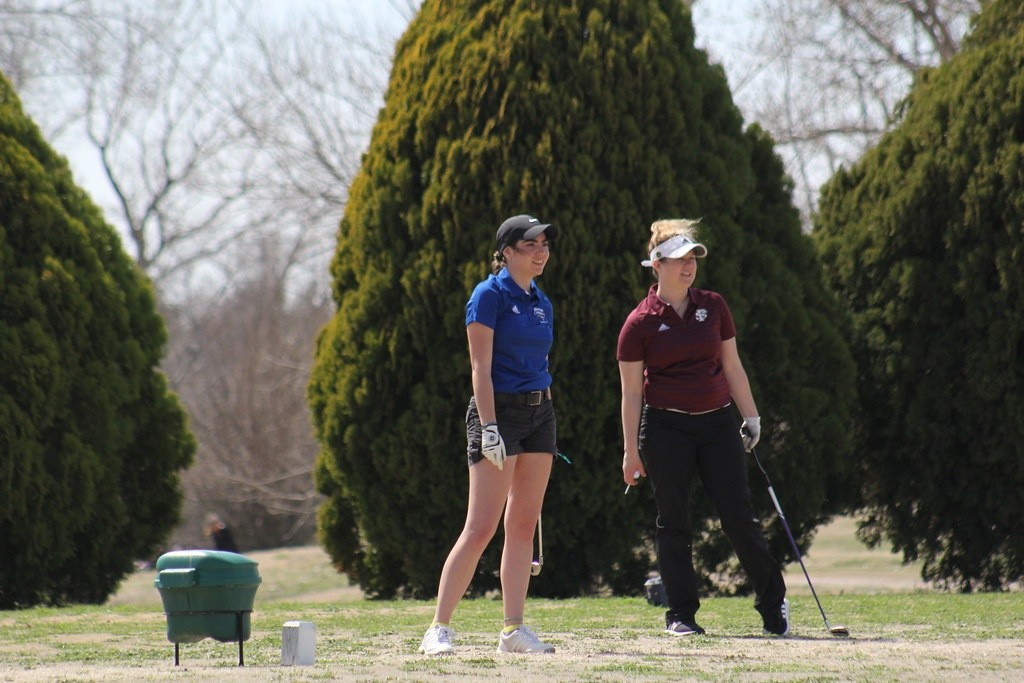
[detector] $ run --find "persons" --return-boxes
[614,218,792,637]
[203,512,241,555]
[414,214,558,657]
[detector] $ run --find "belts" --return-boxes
[494,390,552,406]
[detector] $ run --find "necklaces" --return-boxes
[675,294,689,314]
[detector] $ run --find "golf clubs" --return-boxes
[531,514,543,576]
[741,426,850,637]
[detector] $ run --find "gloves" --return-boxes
[481,422,507,471]
[740,417,763,454]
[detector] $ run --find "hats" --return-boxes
[494,214,559,261]
[640,235,708,269]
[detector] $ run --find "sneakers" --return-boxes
[495,625,555,654]
[665,620,705,636]
[762,598,789,635]
[417,624,455,657]
